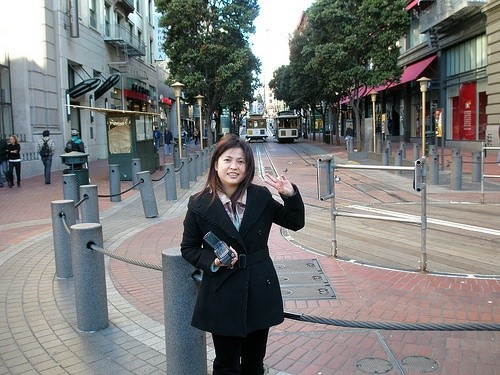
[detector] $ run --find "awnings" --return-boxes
[333,55,437,105]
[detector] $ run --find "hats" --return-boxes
[71,130,79,136]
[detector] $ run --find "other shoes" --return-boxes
[45,181,51,184]
[9,183,13,188]
[17,183,20,187]
[0,183,3,187]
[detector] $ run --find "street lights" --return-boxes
[170,81,185,160]
[416,77,431,156]
[369,89,379,153]
[194,94,205,149]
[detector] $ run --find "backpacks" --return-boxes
[40,138,51,157]
[65,138,79,153]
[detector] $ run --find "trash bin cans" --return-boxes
[326,130,330,144]
[59,151,89,202]
[205,137,207,147]
[174,137,187,168]
[203,138,205,149]
[425,131,435,156]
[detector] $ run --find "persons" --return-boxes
[0,134,9,187]
[344,124,356,154]
[37,130,56,184]
[375,120,379,133]
[163,127,173,156]
[198,130,200,142]
[153,126,162,150]
[178,132,306,375]
[194,128,198,145]
[181,128,188,143]
[8,134,22,188]
[64,130,85,170]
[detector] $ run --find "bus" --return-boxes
[243,112,269,143]
[273,110,301,143]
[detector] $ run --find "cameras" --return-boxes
[203,231,235,264]
